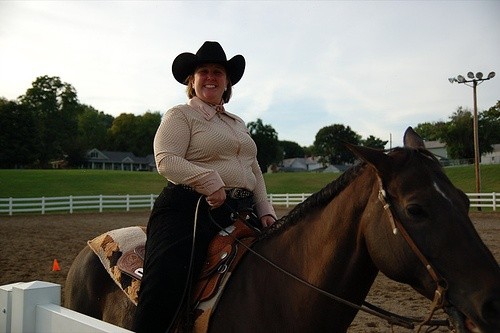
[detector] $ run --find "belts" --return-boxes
[178,182,253,199]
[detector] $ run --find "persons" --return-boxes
[138,40,277,333]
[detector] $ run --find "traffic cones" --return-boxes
[53,258,61,271]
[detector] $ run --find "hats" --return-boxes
[172,41,245,86]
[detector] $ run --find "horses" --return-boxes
[64,125,500,333]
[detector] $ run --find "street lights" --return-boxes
[447,69,495,212]
